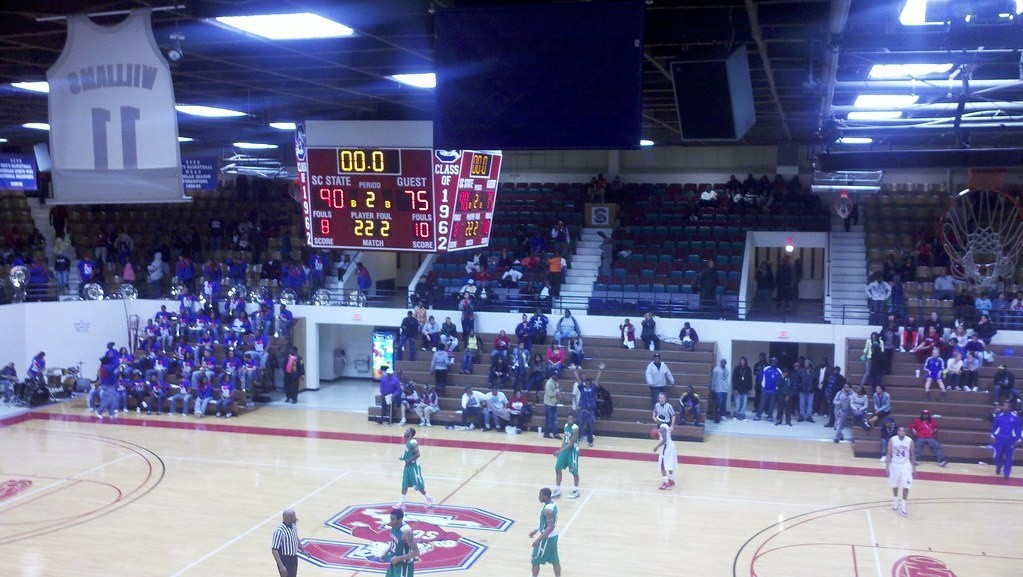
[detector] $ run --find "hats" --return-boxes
[997,364,1006,370]
[770,357,779,363]
[833,366,841,371]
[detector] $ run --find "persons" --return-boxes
[755,256,803,314]
[380,221,700,439]
[843,206,859,232]
[30,352,46,380]
[886,426,915,516]
[551,414,580,497]
[529,488,561,577]
[684,174,818,223]
[392,428,433,508]
[711,349,870,443]
[861,224,1023,478]
[90,275,305,418]
[0,363,19,402]
[379,509,419,577]
[653,415,677,489]
[271,510,311,577]
[0,175,372,304]
[697,260,719,316]
[591,173,624,204]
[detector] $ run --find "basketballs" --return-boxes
[650,429,659,438]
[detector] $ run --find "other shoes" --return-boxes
[940,459,947,466]
[915,460,920,465]
[880,456,887,462]
[715,411,875,445]
[381,418,564,441]
[89,405,235,424]
[996,465,1001,474]
[1005,475,1008,479]
[420,347,506,390]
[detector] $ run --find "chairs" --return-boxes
[0,182,1023,323]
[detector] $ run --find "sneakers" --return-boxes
[669,480,674,485]
[551,489,560,497]
[569,491,579,498]
[925,383,978,395]
[894,502,899,511]
[589,443,593,447]
[392,502,406,509]
[901,507,907,516]
[659,482,671,490]
[425,499,433,508]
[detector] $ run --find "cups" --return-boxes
[450,357,454,364]
[538,426,542,435]
[915,370,921,378]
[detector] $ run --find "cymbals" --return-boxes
[76,362,86,364]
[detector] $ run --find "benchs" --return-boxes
[86,312,1023,463]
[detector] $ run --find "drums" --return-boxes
[48,375,61,388]
[62,366,80,376]
[23,385,50,405]
